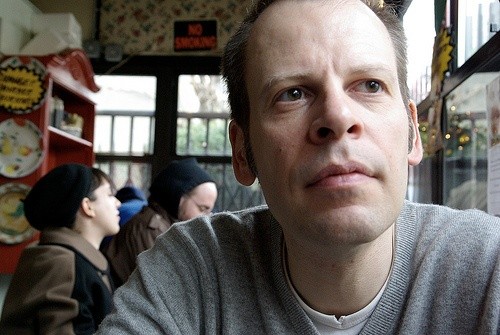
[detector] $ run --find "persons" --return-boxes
[0,164,122,335]
[93,0,500,335]
[105,186,150,243]
[105,157,218,289]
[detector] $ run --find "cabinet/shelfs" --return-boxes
[0,70,97,275]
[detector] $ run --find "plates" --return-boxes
[0,119,47,179]
[0,183,36,244]
[0,56,50,114]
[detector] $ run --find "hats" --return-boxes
[115,186,145,203]
[149,157,215,224]
[19,164,91,230]
